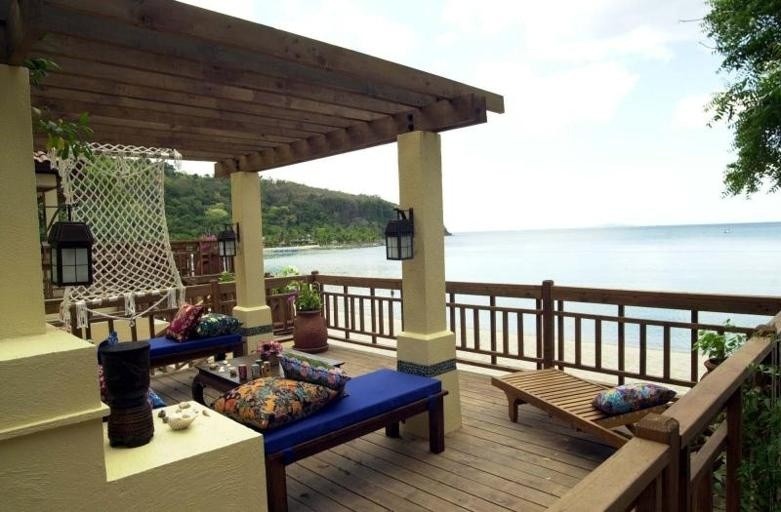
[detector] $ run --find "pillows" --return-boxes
[166,304,204,342]
[283,354,339,369]
[194,313,244,338]
[210,377,338,432]
[276,353,349,396]
[590,380,677,416]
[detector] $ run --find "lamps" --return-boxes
[40,204,94,287]
[217,221,241,257]
[383,207,415,260]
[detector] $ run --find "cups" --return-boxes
[206,354,271,382]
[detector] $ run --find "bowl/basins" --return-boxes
[164,413,198,432]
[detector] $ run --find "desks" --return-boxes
[191,351,345,406]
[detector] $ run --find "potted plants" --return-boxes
[292,284,330,352]
[692,317,749,382]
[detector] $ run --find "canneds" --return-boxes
[238,364,248,384]
[256,358,263,366]
[263,361,273,378]
[251,364,261,380]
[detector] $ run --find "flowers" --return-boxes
[257,338,284,356]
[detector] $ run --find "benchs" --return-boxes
[98,332,245,370]
[260,368,449,512]
[490,368,672,451]
